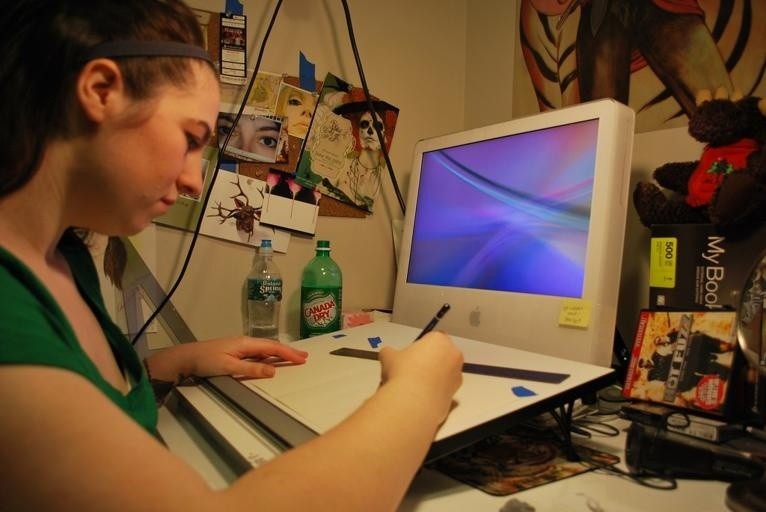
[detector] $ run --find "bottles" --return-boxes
[246,238,284,342]
[298,239,344,339]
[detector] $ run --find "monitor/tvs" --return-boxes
[392,96,636,369]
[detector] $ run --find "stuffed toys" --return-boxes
[632,94,766,236]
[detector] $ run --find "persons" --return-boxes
[0,0,464,511]
[318,108,387,211]
[276,84,318,139]
[636,329,734,390]
[215,110,280,159]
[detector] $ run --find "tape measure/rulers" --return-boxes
[328,346,571,385]
[113,235,198,364]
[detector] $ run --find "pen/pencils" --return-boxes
[414,303,451,342]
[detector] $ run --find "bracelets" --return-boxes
[141,353,151,384]
